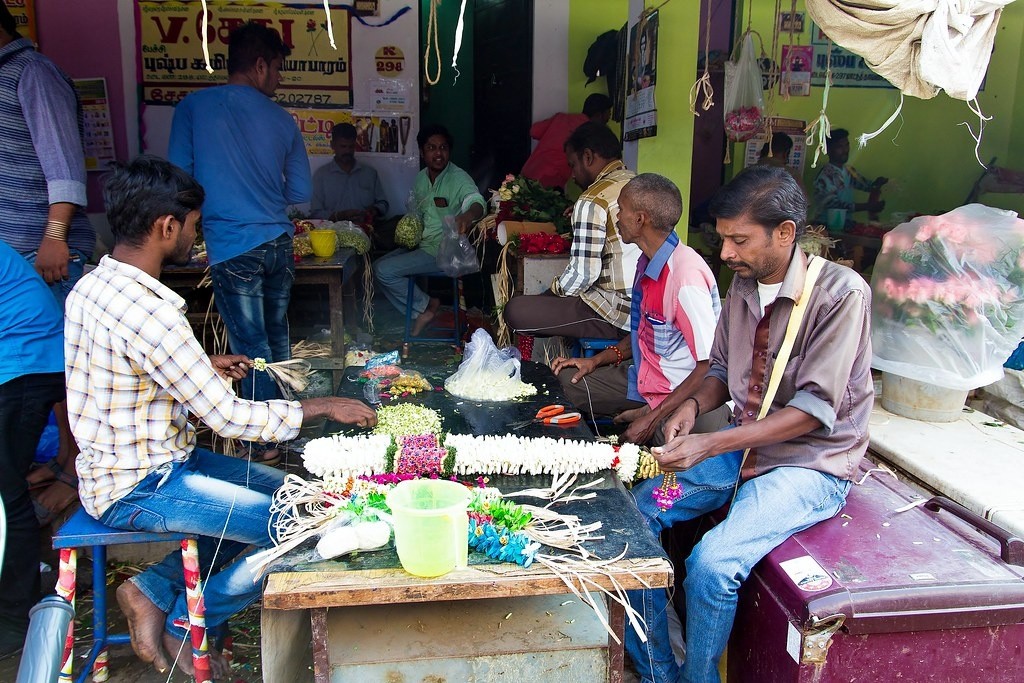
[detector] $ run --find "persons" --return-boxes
[814,129,885,228]
[0,0,97,525]
[551,173,721,446]
[735,132,811,207]
[355,117,398,152]
[0,238,65,659]
[312,122,389,296]
[166,21,313,465]
[371,124,487,337]
[623,163,875,683]
[366,123,374,145]
[520,93,614,189]
[64,153,377,679]
[504,121,644,341]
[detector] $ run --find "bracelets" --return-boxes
[684,397,699,418]
[608,345,623,366]
[44,220,70,241]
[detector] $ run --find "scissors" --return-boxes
[505,405,582,431]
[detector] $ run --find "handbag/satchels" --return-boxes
[444,327,524,403]
[325,220,372,254]
[395,192,423,249]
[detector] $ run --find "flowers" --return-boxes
[252,351,685,567]
[494,175,577,254]
[872,202,1024,338]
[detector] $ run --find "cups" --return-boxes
[827,207,848,230]
[309,229,336,257]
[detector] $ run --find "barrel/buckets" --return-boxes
[386,478,473,578]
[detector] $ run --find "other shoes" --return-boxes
[0,622,28,659]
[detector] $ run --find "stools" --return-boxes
[573,338,622,360]
[50,506,236,683]
[404,272,464,349]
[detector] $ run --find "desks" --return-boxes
[826,230,882,271]
[270,344,674,683]
[160,242,362,361]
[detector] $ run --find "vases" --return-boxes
[880,314,977,422]
